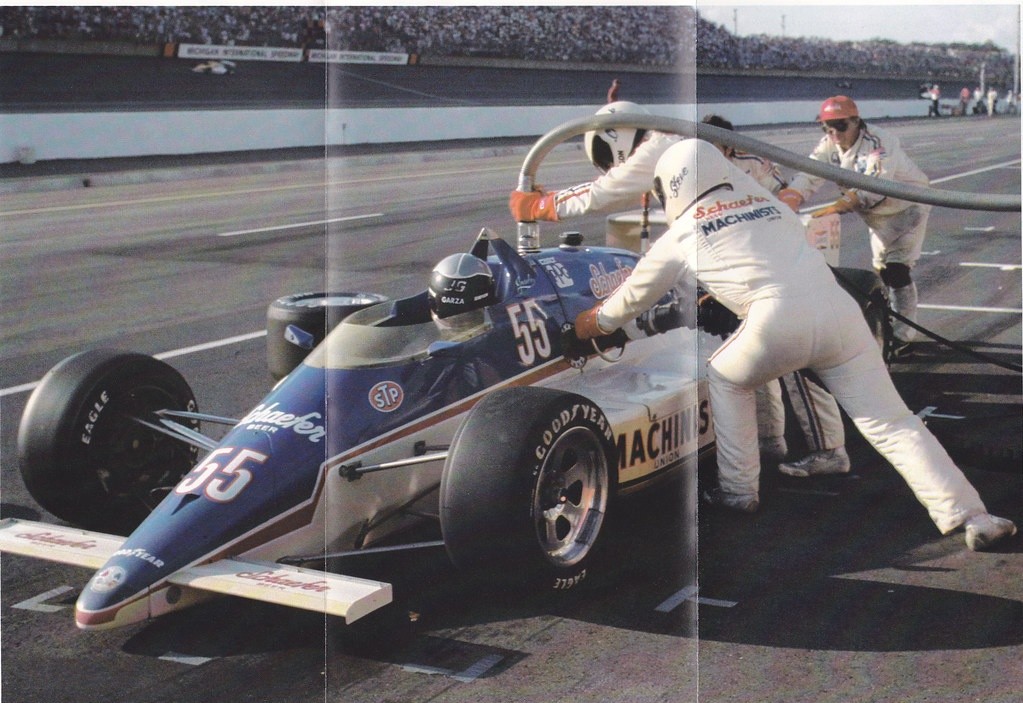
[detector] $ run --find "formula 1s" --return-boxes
[2,229,896,633]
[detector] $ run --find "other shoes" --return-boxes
[705,485,760,515]
[966,517,1017,552]
[886,338,914,361]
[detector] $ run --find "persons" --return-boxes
[973,85,981,106]
[508,79,851,479]
[987,85,998,116]
[776,92,932,360]
[395,252,495,359]
[960,84,971,116]
[698,109,788,199]
[561,138,1018,552]
[0,6,1021,82]
[927,84,945,117]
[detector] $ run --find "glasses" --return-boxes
[826,118,848,132]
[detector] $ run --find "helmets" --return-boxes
[820,95,859,120]
[585,101,651,175]
[654,138,734,225]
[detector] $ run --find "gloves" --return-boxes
[511,185,557,221]
[576,303,614,341]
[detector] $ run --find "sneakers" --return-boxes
[758,438,788,457]
[778,449,849,478]
